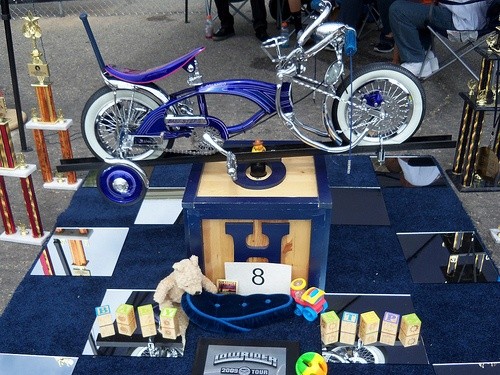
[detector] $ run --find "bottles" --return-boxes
[205,15,213,39]
[281,22,290,48]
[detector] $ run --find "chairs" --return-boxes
[419,0,500,82]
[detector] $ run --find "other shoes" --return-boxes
[324,44,335,51]
[212,24,235,40]
[254,31,276,48]
[304,38,315,47]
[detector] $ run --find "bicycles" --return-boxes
[78,1,427,207]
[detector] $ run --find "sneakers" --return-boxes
[400,59,432,77]
[373,35,396,53]
[426,50,439,72]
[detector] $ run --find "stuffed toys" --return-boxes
[153,255,219,336]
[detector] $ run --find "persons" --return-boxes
[288,0,489,78]
[212,0,273,45]
